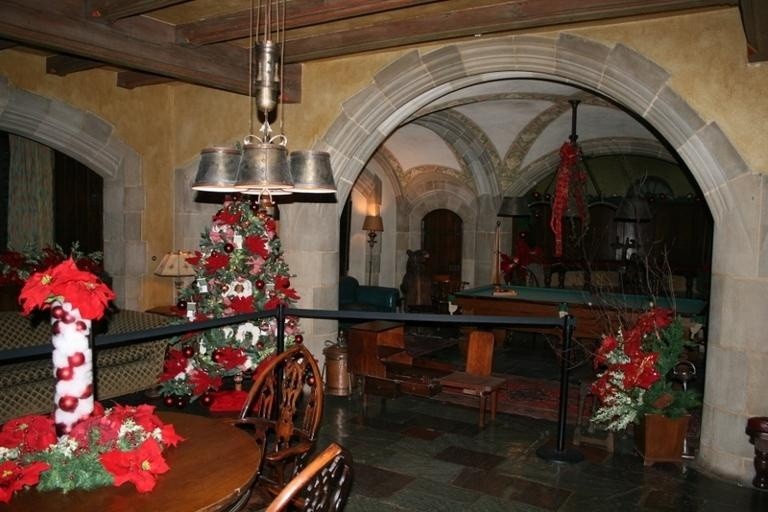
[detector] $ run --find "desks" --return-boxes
[0,412,261,512]
[449,285,707,380]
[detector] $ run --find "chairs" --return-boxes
[339,277,399,328]
[215,343,323,494]
[240,442,353,511]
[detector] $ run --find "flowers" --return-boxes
[586,306,702,432]
[0,398,191,504]
[17,256,115,321]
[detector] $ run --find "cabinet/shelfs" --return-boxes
[346,321,507,428]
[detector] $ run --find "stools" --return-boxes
[433,274,452,303]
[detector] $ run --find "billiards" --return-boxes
[493,286,518,295]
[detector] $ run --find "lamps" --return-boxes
[496,99,652,221]
[153,250,197,307]
[362,215,384,286]
[192,0,337,196]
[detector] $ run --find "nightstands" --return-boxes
[145,306,187,317]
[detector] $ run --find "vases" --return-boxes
[636,414,692,467]
[52,302,95,432]
